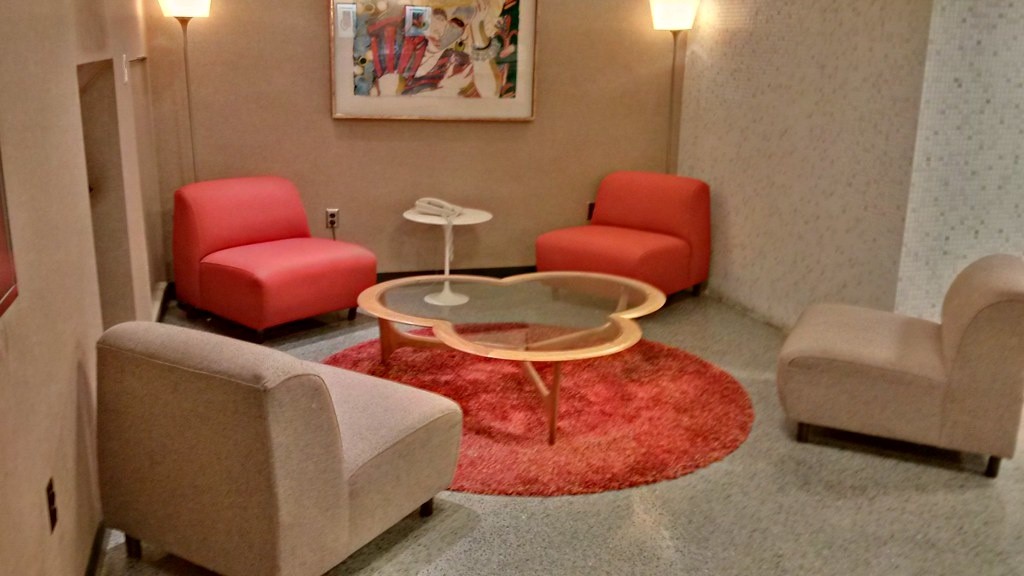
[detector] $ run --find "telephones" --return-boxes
[415,196,463,218]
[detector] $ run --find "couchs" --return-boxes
[535,171,712,307]
[776,253,1023,477]
[96,321,463,576]
[173,176,378,344]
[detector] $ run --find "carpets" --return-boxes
[321,322,755,497]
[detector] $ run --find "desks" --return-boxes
[403,207,492,307]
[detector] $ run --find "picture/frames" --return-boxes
[329,0,541,122]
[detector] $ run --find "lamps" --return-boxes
[648,0,700,175]
[157,0,212,184]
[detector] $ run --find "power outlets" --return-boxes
[326,208,339,229]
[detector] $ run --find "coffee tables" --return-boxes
[358,270,667,446]
